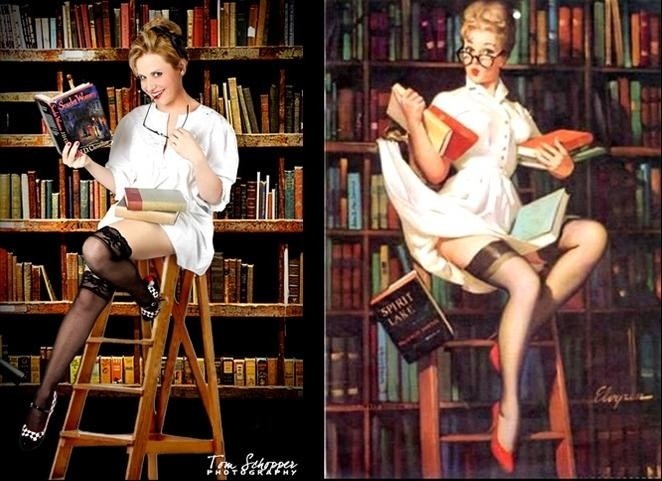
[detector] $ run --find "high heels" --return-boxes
[488,401,515,475]
[487,327,500,373]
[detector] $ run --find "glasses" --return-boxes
[142,99,190,141]
[457,45,508,69]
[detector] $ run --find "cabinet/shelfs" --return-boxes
[0,0,322,481]
[322,0,659,478]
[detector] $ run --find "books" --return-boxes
[0,1,662,481]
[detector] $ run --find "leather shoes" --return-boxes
[140,276,161,321]
[20,391,58,451]
